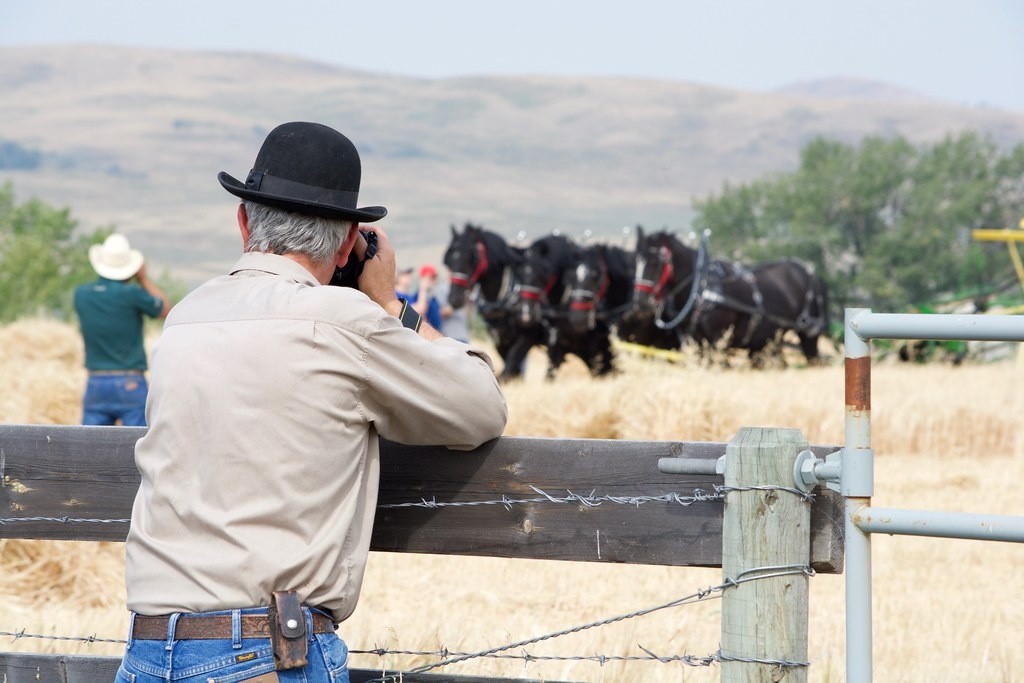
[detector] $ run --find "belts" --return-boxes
[133,610,335,639]
[91,369,142,376]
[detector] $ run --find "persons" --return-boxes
[117,121,508,683]
[396,264,469,346]
[74,233,167,426]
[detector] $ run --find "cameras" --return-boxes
[327,230,369,290]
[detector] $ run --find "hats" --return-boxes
[399,267,413,275]
[88,233,143,281]
[420,265,437,279]
[217,121,387,222]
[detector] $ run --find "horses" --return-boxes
[442,222,831,383]
